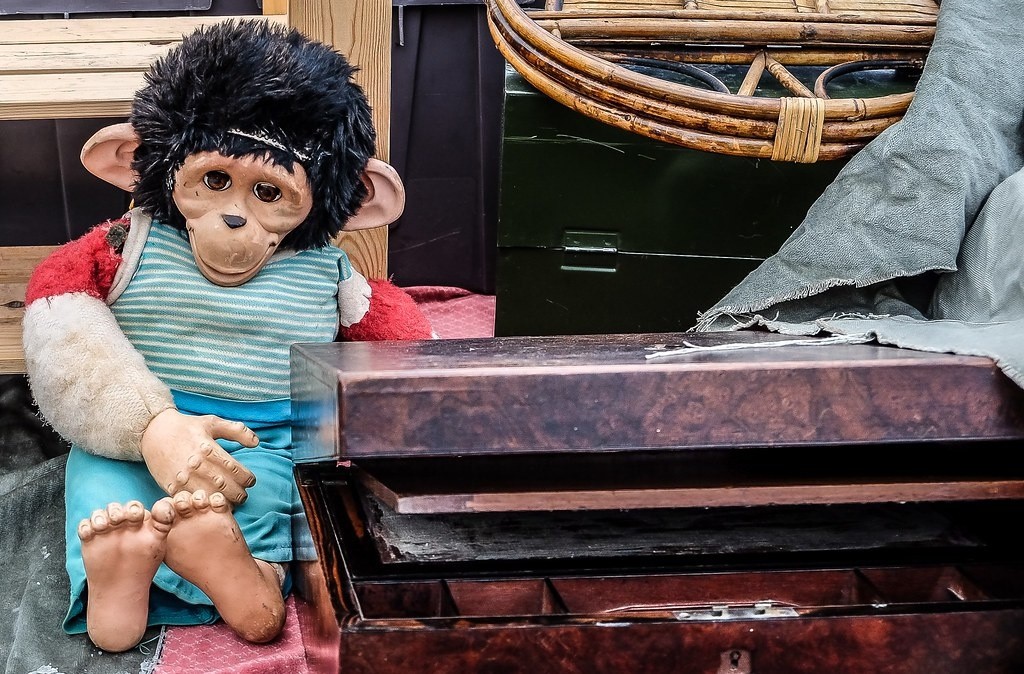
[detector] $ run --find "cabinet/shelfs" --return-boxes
[0,0,392,383]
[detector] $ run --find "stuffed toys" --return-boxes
[21,19,438,653]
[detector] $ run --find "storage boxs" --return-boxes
[495,57,938,338]
